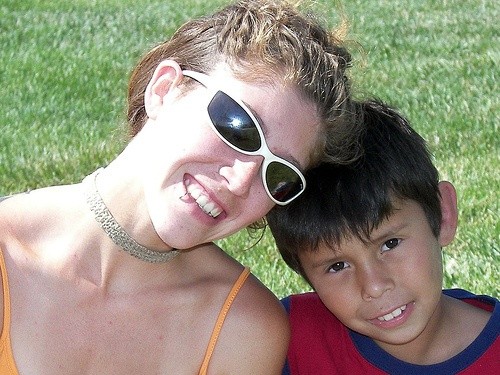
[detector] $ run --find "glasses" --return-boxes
[183,70,308,204]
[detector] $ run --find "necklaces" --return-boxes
[82,167,184,264]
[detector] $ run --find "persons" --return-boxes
[1,1,373,375]
[265,91,500,374]
